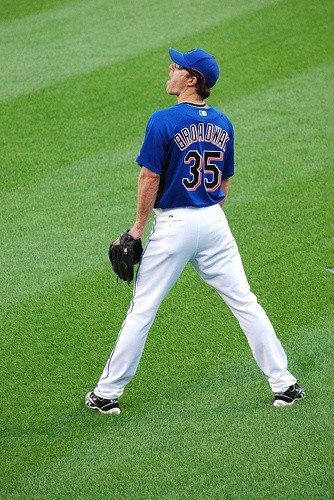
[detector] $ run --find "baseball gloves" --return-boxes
[109,230,144,287]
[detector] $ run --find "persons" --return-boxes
[84,49,308,416]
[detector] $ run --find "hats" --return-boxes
[169,48,219,88]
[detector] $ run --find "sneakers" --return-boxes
[273,383,306,407]
[85,392,121,414]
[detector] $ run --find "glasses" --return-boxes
[175,63,199,81]
